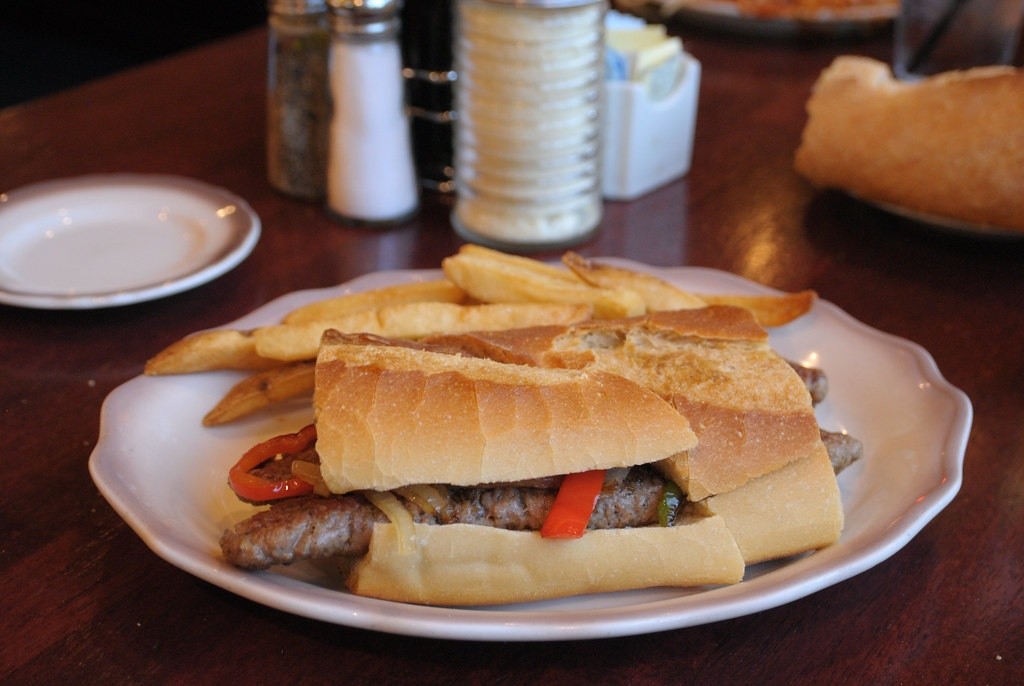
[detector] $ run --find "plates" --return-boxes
[87,255,973,640]
[846,191,1023,241]
[0,170,262,312]
[659,0,893,38]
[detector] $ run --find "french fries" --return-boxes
[143,242,815,427]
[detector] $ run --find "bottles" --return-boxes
[263,1,423,230]
[450,1,612,256]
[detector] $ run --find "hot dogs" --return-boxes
[220,304,844,606]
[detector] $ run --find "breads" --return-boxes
[796,56,1023,238]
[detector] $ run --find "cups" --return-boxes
[891,0,1024,84]
